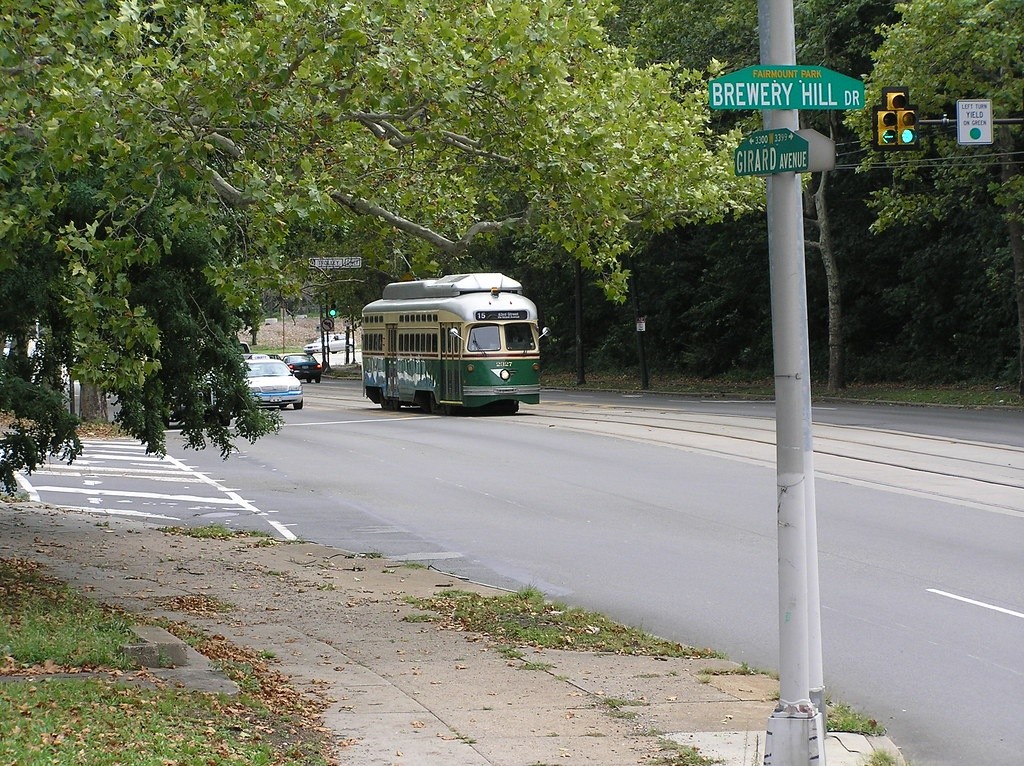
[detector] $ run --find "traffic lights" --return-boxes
[876,111,898,146]
[886,92,907,112]
[896,109,918,146]
[329,303,338,318]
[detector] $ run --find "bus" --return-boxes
[360,272,552,417]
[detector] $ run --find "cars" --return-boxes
[282,354,323,384]
[237,342,283,361]
[303,333,357,357]
[238,359,304,410]
